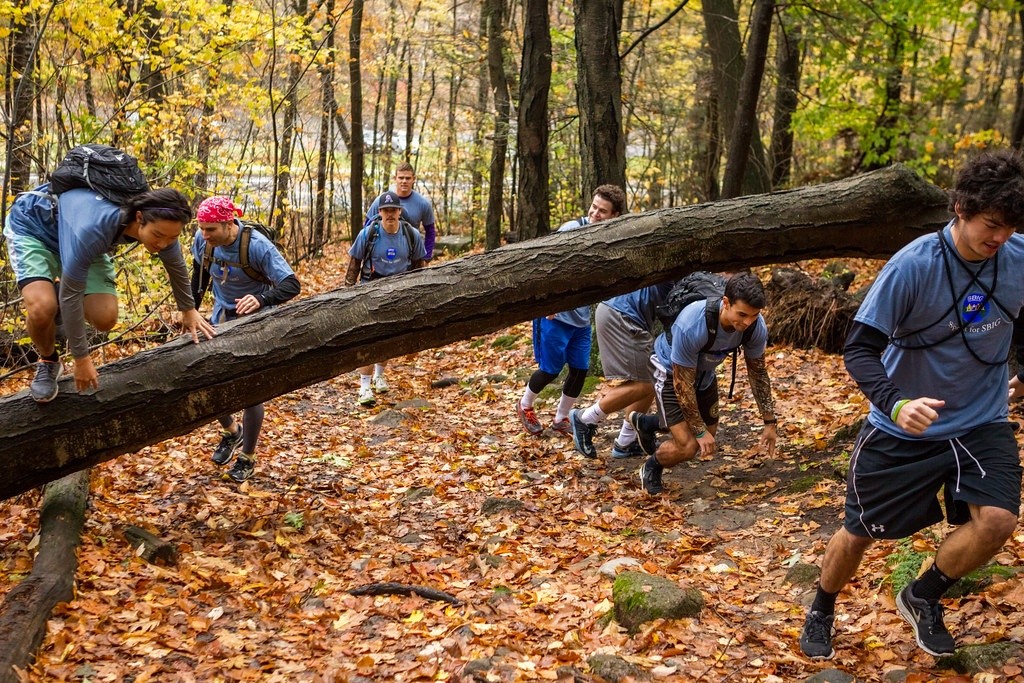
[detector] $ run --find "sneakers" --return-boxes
[53,277,67,339]
[800,606,834,661]
[568,407,598,461]
[551,416,574,442]
[612,436,643,459]
[221,453,256,483]
[639,458,664,496]
[518,398,544,436]
[358,387,377,405]
[895,581,956,656]
[30,353,63,403]
[210,423,243,465]
[629,409,658,455]
[372,375,390,394]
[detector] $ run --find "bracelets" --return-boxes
[764,419,777,424]
[892,400,911,423]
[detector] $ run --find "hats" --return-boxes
[377,191,403,209]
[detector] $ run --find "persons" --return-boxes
[519,184,626,441]
[190,197,302,482]
[345,191,427,405]
[364,162,435,267]
[569,282,674,460]
[799,146,1024,660]
[630,272,777,496]
[2,182,217,403]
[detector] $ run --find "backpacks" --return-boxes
[50,144,149,250]
[202,219,284,286]
[655,270,758,354]
